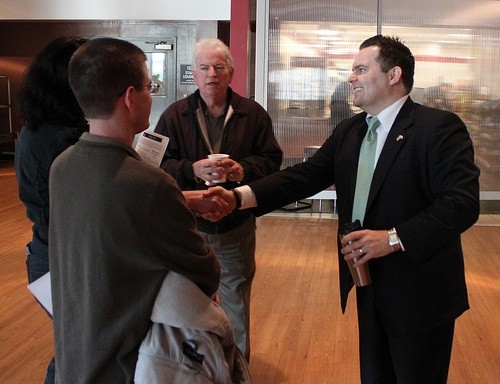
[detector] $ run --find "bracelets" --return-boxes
[231,188,241,209]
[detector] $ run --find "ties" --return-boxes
[352,117,380,227]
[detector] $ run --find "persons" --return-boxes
[154,39,284,367]
[13,37,92,384]
[152,74,166,96]
[424,82,452,113]
[195,34,482,384]
[329,81,355,131]
[49,37,221,384]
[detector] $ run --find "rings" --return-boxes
[360,249,363,252]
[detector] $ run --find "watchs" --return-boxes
[387,228,402,252]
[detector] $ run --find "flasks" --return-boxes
[339,220,372,287]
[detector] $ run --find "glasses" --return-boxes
[132,81,160,93]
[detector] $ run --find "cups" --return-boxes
[207,153,230,183]
